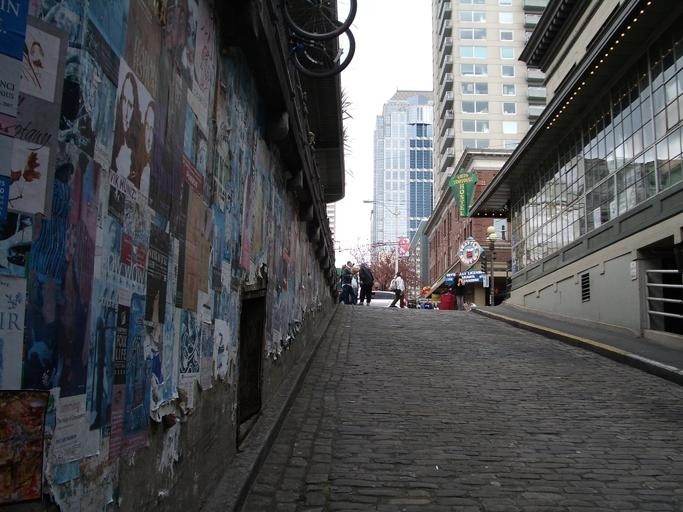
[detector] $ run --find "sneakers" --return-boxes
[367,303,370,306]
[359,302,363,305]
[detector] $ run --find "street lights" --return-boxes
[484,225,500,306]
[364,198,402,291]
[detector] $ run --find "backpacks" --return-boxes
[389,278,397,290]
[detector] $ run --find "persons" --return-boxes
[452,272,465,311]
[389,271,406,309]
[423,299,432,309]
[339,261,374,307]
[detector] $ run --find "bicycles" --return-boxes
[282,0,358,80]
